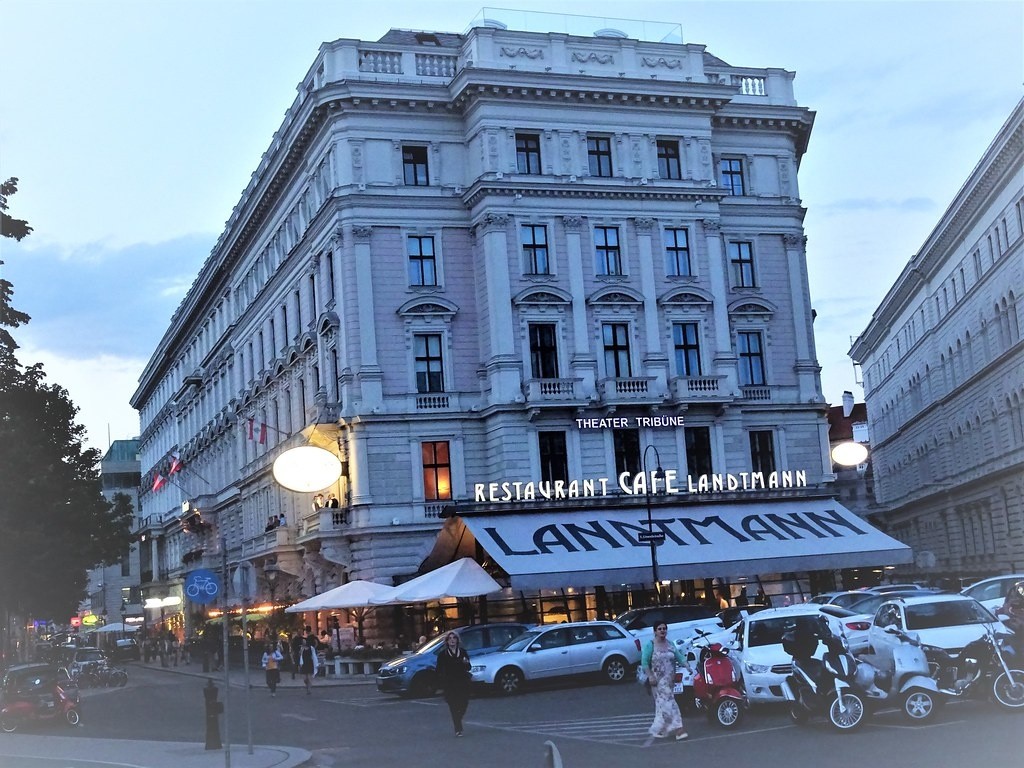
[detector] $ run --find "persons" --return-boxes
[435,632,473,737]
[262,626,438,698]
[263,514,287,532]
[648,589,770,610]
[641,620,693,741]
[142,633,225,672]
[311,493,338,522]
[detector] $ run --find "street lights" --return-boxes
[643,444,665,606]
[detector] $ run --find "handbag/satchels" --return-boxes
[636,665,650,685]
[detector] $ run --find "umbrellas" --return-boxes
[368,557,504,625]
[285,580,413,641]
[95,623,138,632]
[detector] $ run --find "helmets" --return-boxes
[874,670,892,692]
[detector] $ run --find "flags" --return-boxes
[151,472,168,492]
[247,418,266,445]
[168,456,183,477]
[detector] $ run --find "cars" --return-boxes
[373,574,1024,732]
[32,642,107,674]
[112,638,140,660]
[2,662,79,707]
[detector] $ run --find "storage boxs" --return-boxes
[782,630,820,660]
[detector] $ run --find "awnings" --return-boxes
[461,496,914,593]
[204,613,266,626]
[146,613,180,625]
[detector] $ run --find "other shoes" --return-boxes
[676,732,689,741]
[456,729,464,736]
[649,730,665,739]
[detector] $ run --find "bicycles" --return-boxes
[87,662,128,688]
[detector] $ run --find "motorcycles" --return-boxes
[691,628,744,728]
[0,675,81,733]
[921,617,1024,711]
[856,627,938,723]
[780,614,867,731]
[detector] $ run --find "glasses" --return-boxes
[657,627,667,632]
